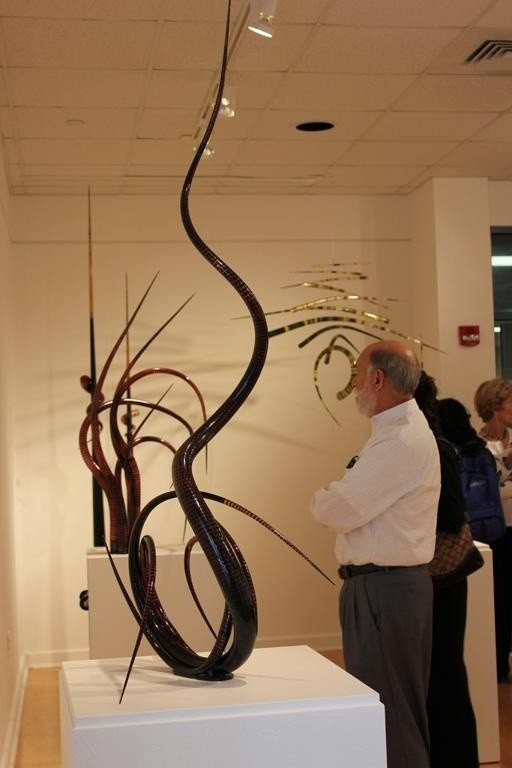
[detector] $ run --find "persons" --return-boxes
[308,341,442,768]
[475,379,512,682]
[413,376,479,768]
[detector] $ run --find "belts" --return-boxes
[337,563,405,579]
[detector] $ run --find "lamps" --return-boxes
[192,0,281,159]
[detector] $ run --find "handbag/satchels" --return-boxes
[428,519,485,586]
